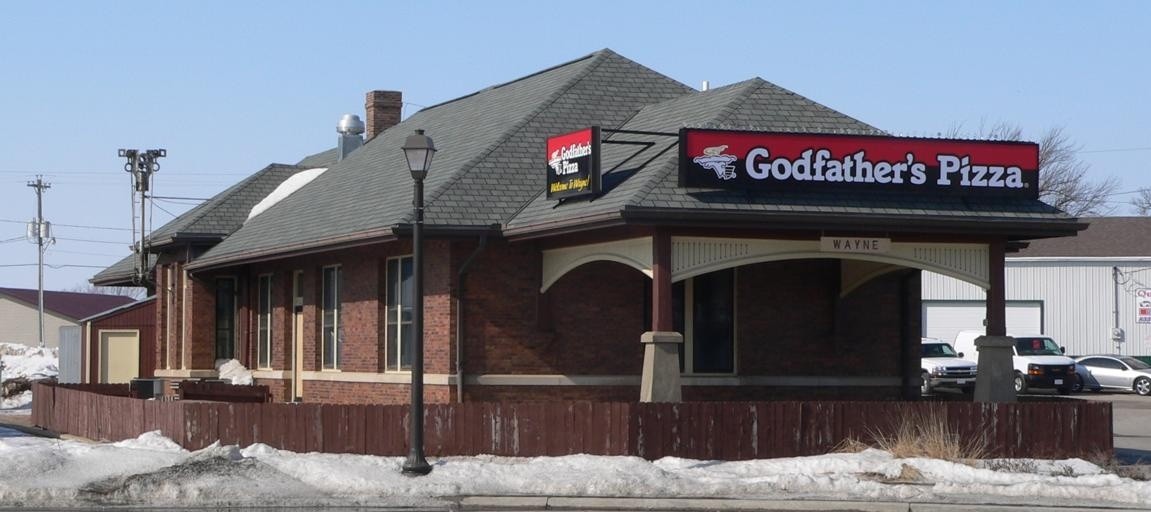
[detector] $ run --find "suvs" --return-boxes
[920,337,978,397]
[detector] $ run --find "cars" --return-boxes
[1070,353,1150,397]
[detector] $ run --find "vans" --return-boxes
[955,330,1076,395]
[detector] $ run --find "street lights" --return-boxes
[400,129,437,477]
